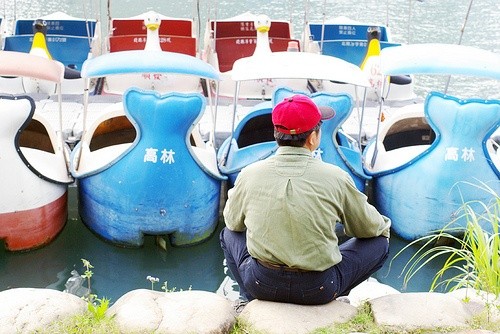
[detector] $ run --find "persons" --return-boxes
[219,94,392,306]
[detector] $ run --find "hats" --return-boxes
[272,95,335,134]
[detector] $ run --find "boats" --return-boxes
[69,48,228,248]
[0,50,101,253]
[204,11,309,106]
[217,51,372,236]
[299,15,418,104]
[96,9,204,97]
[362,44,499,245]
[0,11,102,95]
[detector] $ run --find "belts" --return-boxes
[256,259,311,272]
